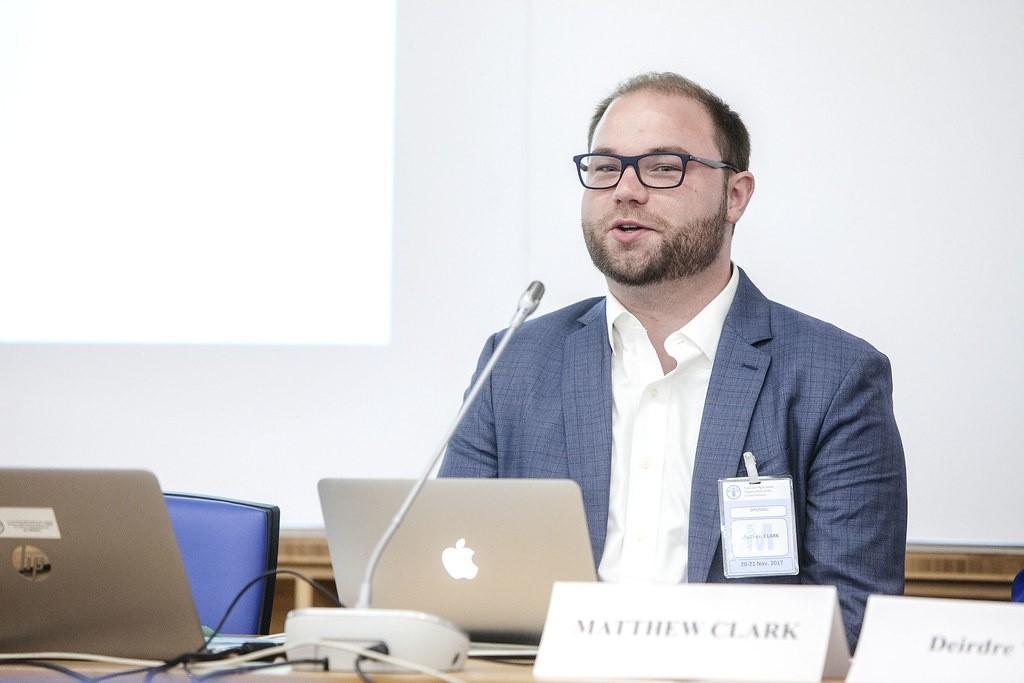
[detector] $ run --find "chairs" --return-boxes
[162,490,281,635]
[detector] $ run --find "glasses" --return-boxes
[572,151,742,191]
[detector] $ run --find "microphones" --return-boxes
[286,281,546,675]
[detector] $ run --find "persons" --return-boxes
[436,72,908,652]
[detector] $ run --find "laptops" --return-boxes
[0,467,286,667]
[315,478,598,648]
